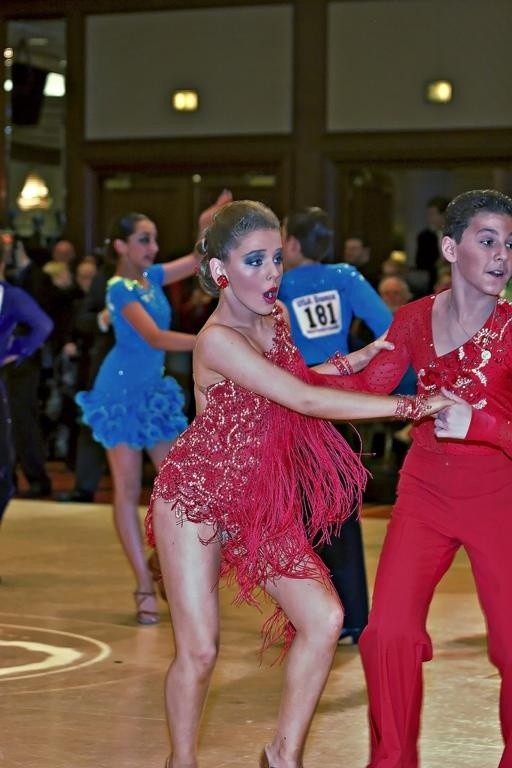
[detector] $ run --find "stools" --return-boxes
[364,427,397,472]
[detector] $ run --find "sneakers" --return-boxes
[16,482,54,499]
[57,491,97,503]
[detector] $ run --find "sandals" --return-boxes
[147,555,168,602]
[134,587,160,625]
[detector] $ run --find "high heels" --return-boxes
[338,624,367,645]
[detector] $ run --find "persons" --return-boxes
[208,206,414,646]
[299,187,512,767]
[72,188,233,624]
[342,196,453,495]
[0,233,217,503]
[145,201,456,767]
